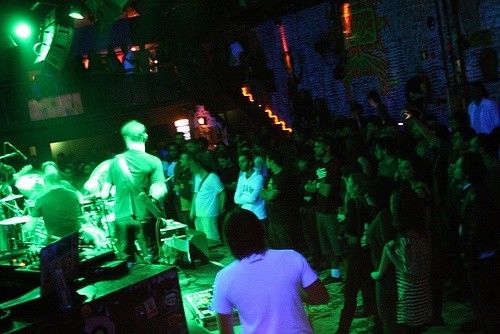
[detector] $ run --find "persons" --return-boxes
[0,163,29,250]
[26,161,85,246]
[99,120,168,264]
[57,144,144,262]
[146,80,500,334]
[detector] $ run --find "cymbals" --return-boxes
[0,194,24,203]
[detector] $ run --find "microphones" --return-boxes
[6,142,28,160]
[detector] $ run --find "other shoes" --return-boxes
[355,305,365,311]
[355,311,378,317]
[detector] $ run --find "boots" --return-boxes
[335,309,354,334]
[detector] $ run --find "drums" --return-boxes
[0,216,31,263]
[78,195,103,218]
[82,192,110,222]
[20,225,48,243]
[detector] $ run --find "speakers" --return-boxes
[34,5,74,74]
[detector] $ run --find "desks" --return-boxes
[160,220,192,266]
[0,250,189,334]
